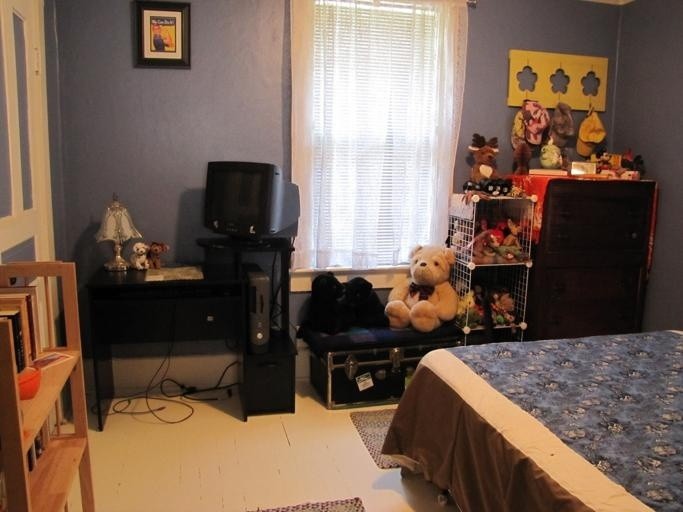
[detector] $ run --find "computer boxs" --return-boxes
[244,269,272,360]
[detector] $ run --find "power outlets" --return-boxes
[295,325,310,347]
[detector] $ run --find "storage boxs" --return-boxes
[310,341,456,408]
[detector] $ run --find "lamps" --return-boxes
[94,192,143,271]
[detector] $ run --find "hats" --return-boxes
[577,107,606,157]
[511,110,539,151]
[548,103,574,146]
[522,100,550,145]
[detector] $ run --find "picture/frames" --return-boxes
[133,0,190,69]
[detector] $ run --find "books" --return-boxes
[0,284,41,374]
[27,420,50,472]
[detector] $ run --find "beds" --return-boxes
[382,331,683,512]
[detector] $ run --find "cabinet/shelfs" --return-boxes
[506,173,659,340]
[0,261,95,512]
[450,194,538,345]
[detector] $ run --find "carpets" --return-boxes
[350,409,400,468]
[247,497,365,512]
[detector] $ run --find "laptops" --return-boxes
[201,160,301,237]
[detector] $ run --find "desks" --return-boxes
[76,240,298,432]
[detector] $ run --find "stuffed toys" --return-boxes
[130,242,149,270]
[384,243,459,334]
[465,132,502,185]
[472,218,525,267]
[458,284,514,327]
[294,270,391,333]
[149,240,166,269]
